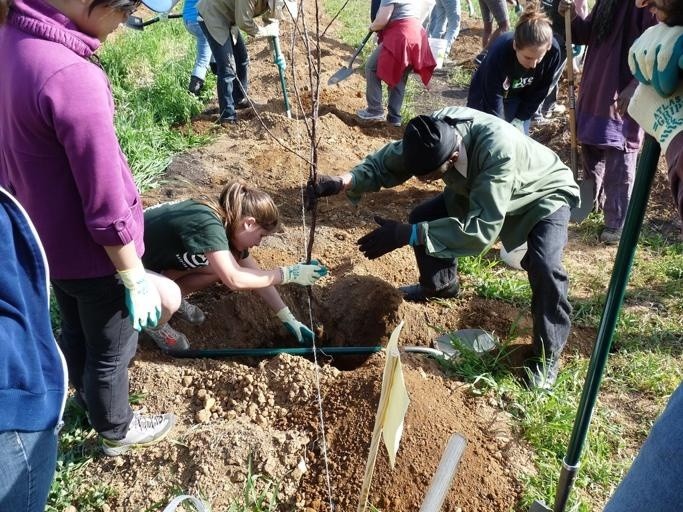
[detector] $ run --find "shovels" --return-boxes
[565,0,594,222]
[328,32,373,84]
[166,329,500,368]
[530,132,661,512]
[123,15,183,31]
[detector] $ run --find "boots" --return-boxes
[210,61,218,76]
[188,75,205,97]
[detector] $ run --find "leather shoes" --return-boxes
[400,284,461,303]
[528,356,557,402]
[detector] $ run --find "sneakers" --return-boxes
[473,53,487,64]
[601,226,622,244]
[356,106,386,123]
[235,99,255,109]
[173,298,208,324]
[385,118,402,128]
[146,320,191,354]
[542,103,566,118]
[102,413,175,457]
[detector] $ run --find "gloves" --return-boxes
[303,173,347,211]
[156,12,170,23]
[276,306,317,343]
[628,22,683,99]
[117,261,163,331]
[272,50,288,71]
[356,215,410,260]
[259,23,281,38]
[627,77,683,156]
[279,259,328,287]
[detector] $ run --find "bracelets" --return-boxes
[569,11,579,23]
[279,268,285,285]
[340,176,345,193]
[410,221,420,247]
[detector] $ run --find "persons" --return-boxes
[1,187,70,512]
[355,0,436,127]
[559,0,659,243]
[466,1,561,138]
[303,106,582,390]
[183,0,217,93]
[1,1,177,457]
[538,1,589,76]
[601,1,682,510]
[141,179,328,352]
[474,0,510,64]
[194,1,298,124]
[422,0,462,75]
